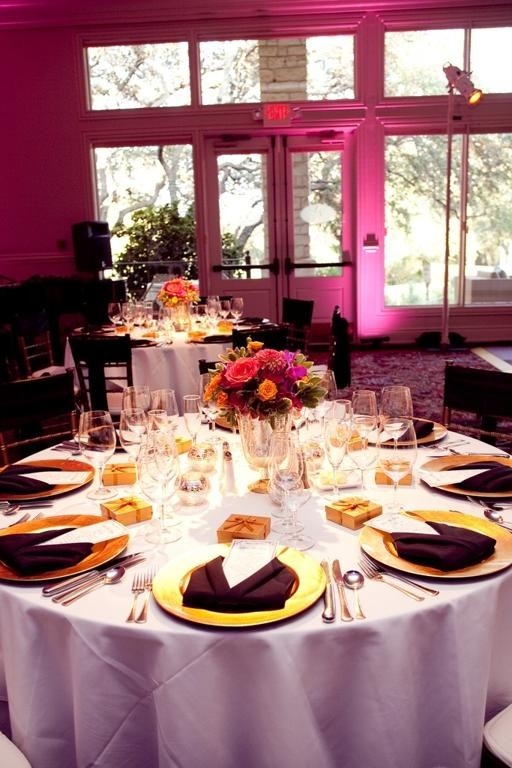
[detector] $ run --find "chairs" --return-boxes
[281,296,314,329]
[198,359,227,407]
[192,296,234,319]
[231,328,287,353]
[67,330,153,417]
[58,313,88,352]
[17,330,55,376]
[0,368,78,465]
[441,358,511,456]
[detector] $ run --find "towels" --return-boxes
[447,460,512,492]
[182,555,296,610]
[0,463,62,494]
[391,520,496,571]
[0,528,94,577]
[372,416,434,443]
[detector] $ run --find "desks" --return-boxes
[0,410,512,768]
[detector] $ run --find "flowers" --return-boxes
[156,278,201,313]
[198,336,328,429]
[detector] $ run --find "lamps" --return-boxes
[442,60,484,348]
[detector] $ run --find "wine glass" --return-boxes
[310,371,337,434]
[116,385,203,543]
[324,419,349,503]
[77,411,118,501]
[108,296,243,338]
[267,421,317,550]
[346,418,381,498]
[375,418,418,522]
[378,386,416,453]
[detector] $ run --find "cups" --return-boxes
[331,398,352,422]
[351,389,377,449]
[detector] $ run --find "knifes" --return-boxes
[52,558,146,604]
[331,559,354,621]
[42,552,141,595]
[319,560,336,623]
[2,503,53,508]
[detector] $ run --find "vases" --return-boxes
[171,306,190,327]
[232,406,293,494]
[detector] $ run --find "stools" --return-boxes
[0,732,33,768]
[481,703,512,767]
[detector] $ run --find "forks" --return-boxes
[356,558,425,602]
[360,552,440,597]
[135,565,160,624]
[126,572,146,623]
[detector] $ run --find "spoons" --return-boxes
[60,565,125,605]
[343,570,366,620]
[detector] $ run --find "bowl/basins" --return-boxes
[246,481,275,499]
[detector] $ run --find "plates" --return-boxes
[359,510,511,579]
[310,472,361,493]
[0,514,130,582]
[151,545,328,629]
[1,459,95,501]
[418,453,511,500]
[358,418,448,448]
